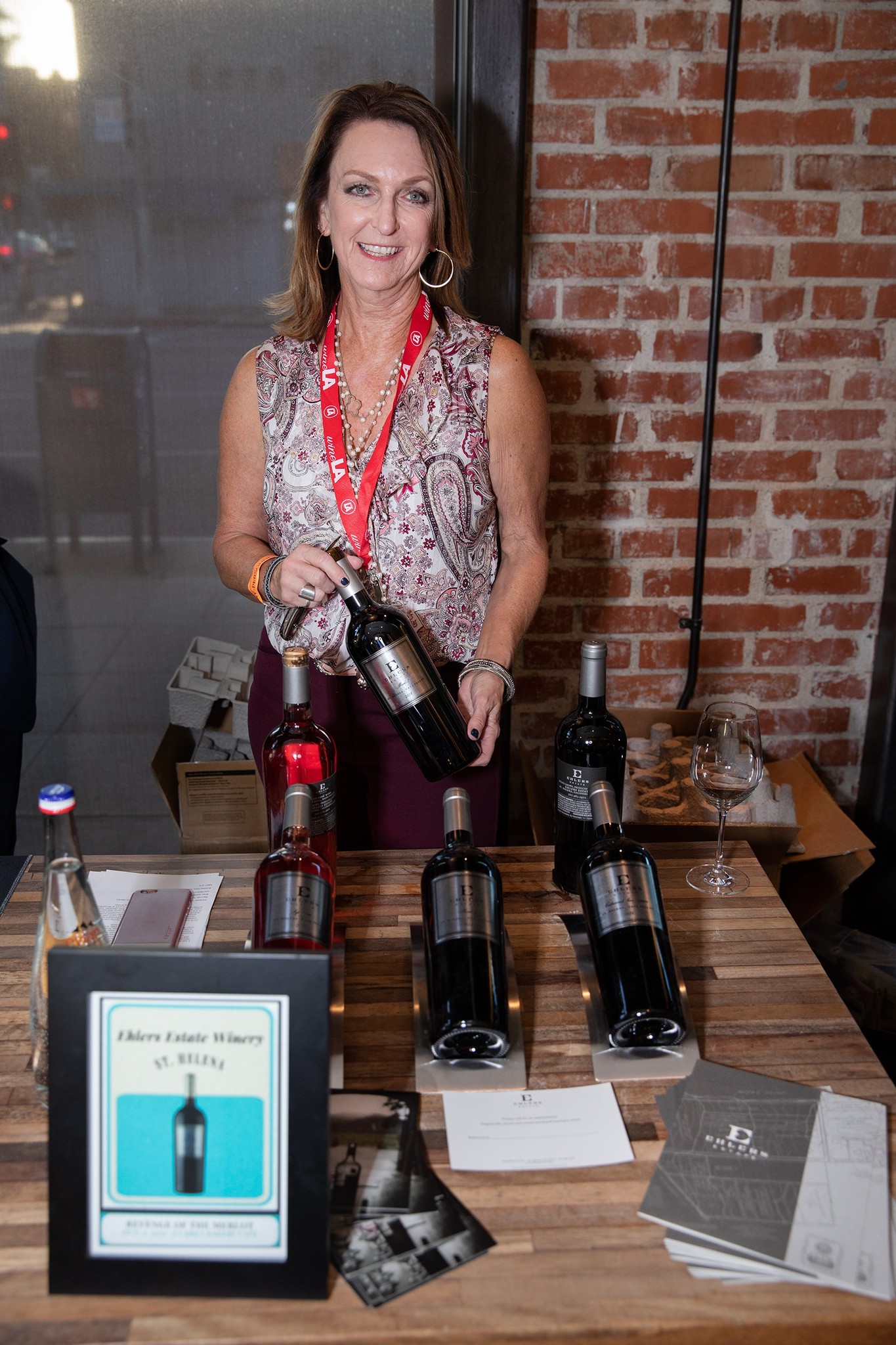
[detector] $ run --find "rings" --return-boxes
[303,600,311,607]
[299,582,315,601]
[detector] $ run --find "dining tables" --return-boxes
[0,839,896,1344]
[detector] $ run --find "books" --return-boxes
[328,1087,498,1308]
[638,1056,896,1306]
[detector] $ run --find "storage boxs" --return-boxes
[518,706,878,930]
[149,723,272,854]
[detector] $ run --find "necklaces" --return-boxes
[334,312,408,475]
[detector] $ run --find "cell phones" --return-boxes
[111,887,194,950]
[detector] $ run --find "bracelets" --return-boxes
[247,555,290,609]
[457,659,516,703]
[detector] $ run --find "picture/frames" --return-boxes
[43,943,329,1299]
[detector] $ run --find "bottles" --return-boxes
[421,787,510,1058]
[325,546,482,782]
[29,783,109,1111]
[254,647,338,952]
[553,640,688,1047]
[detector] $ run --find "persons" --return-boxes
[0,537,40,855]
[210,81,554,845]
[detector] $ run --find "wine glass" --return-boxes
[686,701,763,895]
